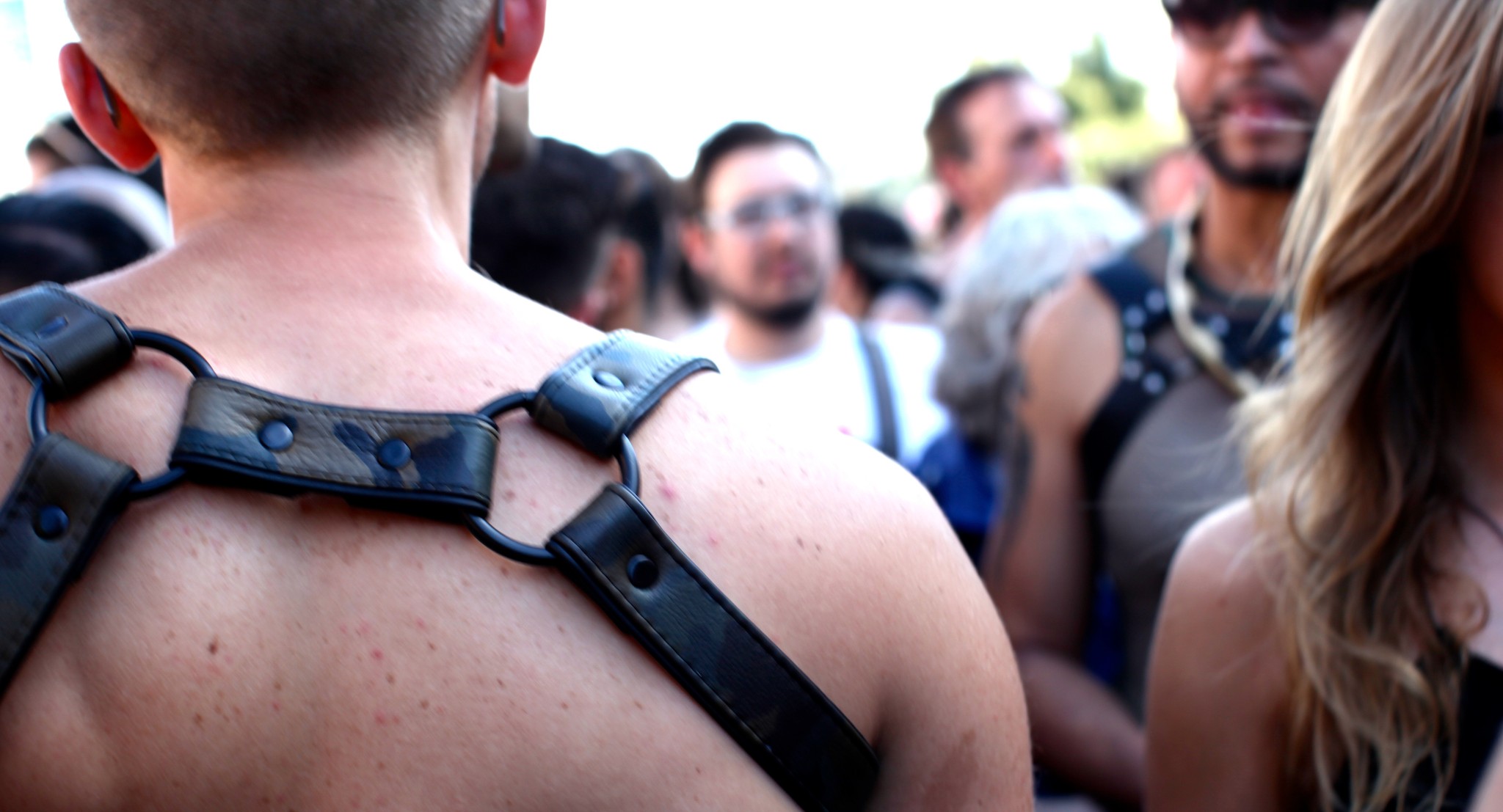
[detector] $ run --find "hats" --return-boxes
[837,201,944,305]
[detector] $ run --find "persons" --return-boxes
[656,122,960,473]
[2,0,1503,812]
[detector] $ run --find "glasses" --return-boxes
[1159,1,1348,40]
